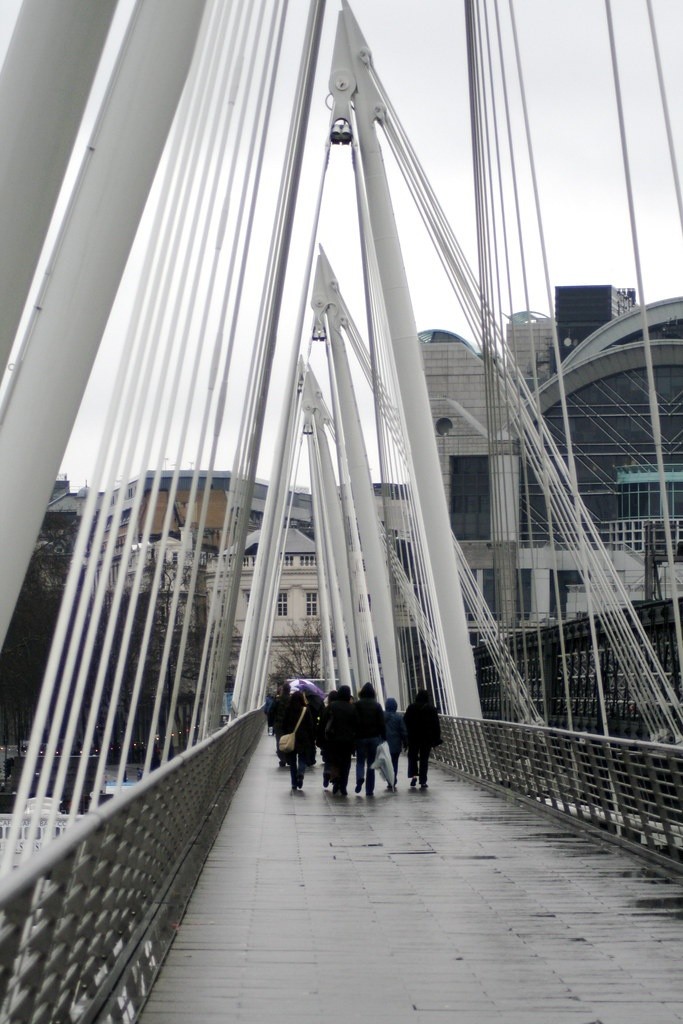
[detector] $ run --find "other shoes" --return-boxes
[323,771,330,788]
[421,784,428,788]
[296,771,305,788]
[355,779,365,793]
[340,785,348,796]
[411,777,417,787]
[333,785,340,794]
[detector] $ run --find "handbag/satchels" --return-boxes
[279,733,295,752]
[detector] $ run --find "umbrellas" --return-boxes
[287,677,325,700]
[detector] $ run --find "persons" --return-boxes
[351,682,387,796]
[402,690,443,788]
[264,680,354,796]
[381,699,408,792]
